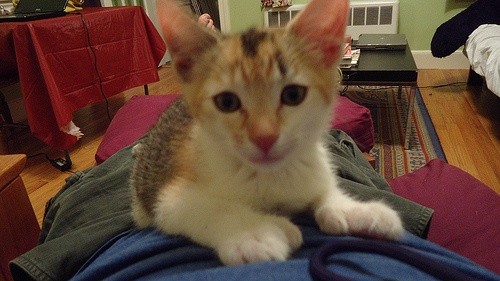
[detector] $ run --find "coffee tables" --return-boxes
[340,40,418,149]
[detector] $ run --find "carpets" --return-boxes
[340,83,449,181]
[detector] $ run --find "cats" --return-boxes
[128,0,404,265]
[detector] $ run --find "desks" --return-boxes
[0,6,166,165]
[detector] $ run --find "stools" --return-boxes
[94,95,374,166]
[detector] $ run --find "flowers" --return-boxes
[259,0,292,13]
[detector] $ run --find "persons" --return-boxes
[8,13,500,281]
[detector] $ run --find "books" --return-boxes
[340,42,360,68]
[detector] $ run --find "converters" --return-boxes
[52,158,71,171]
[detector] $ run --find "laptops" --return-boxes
[356,33,407,51]
[0,0,68,21]
